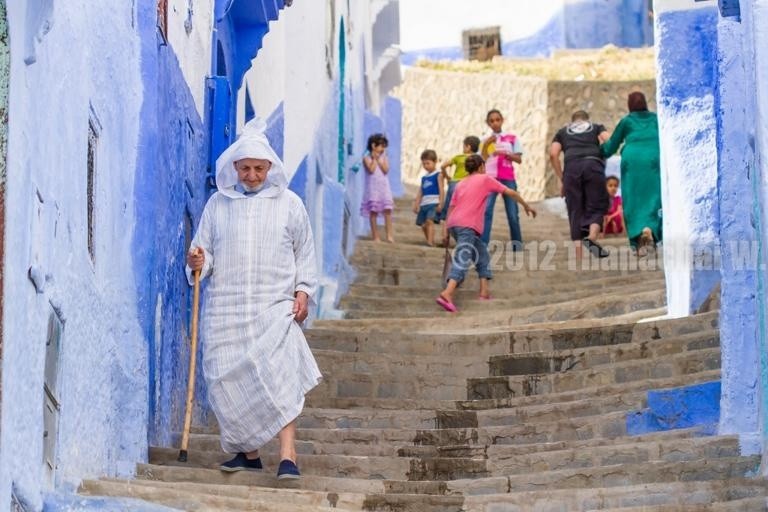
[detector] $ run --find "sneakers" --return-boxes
[220,452,263,472]
[277,459,300,481]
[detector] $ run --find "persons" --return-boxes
[479,109,526,251]
[600,176,624,239]
[435,153,536,313]
[550,111,612,261]
[362,134,395,244]
[413,150,445,247]
[184,118,318,482]
[597,91,661,258]
[437,136,480,247]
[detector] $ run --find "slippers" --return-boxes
[636,234,649,257]
[647,240,657,254]
[583,238,609,259]
[479,294,493,300]
[436,297,456,314]
